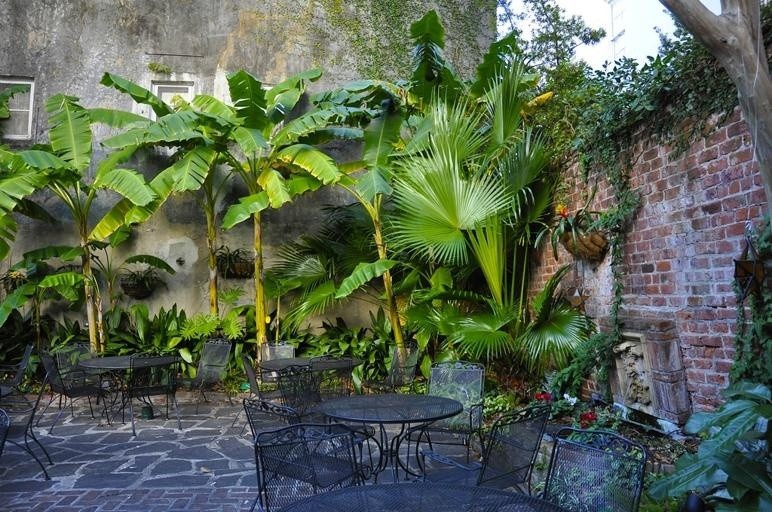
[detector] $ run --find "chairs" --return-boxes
[36,338,233,437]
[362,342,418,395]
[405,361,487,481]
[1,344,53,481]
[243,397,372,510]
[231,340,362,436]
[419,403,551,496]
[253,422,368,510]
[543,425,647,511]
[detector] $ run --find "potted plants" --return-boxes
[110,265,170,300]
[202,244,255,282]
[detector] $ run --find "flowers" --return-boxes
[530,179,606,261]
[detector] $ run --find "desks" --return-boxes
[272,482,572,511]
[321,393,463,483]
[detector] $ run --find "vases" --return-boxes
[561,232,605,261]
